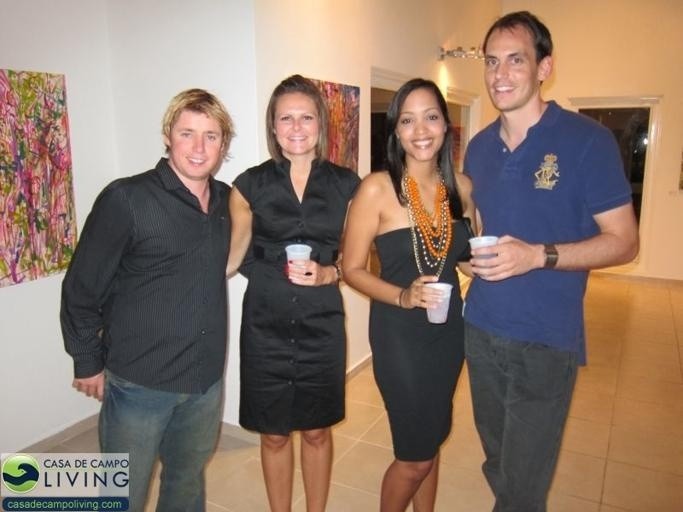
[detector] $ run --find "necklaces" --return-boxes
[403,162,452,282]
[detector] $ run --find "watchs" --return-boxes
[332,263,342,281]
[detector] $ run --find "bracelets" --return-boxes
[542,243,559,269]
[398,288,405,308]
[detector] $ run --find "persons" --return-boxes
[462,10,641,512]
[59,88,256,512]
[340,77,482,512]
[230,74,363,512]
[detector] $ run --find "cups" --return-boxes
[469,236,500,277]
[285,244,312,276]
[424,282,453,324]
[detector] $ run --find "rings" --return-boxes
[304,272,312,276]
